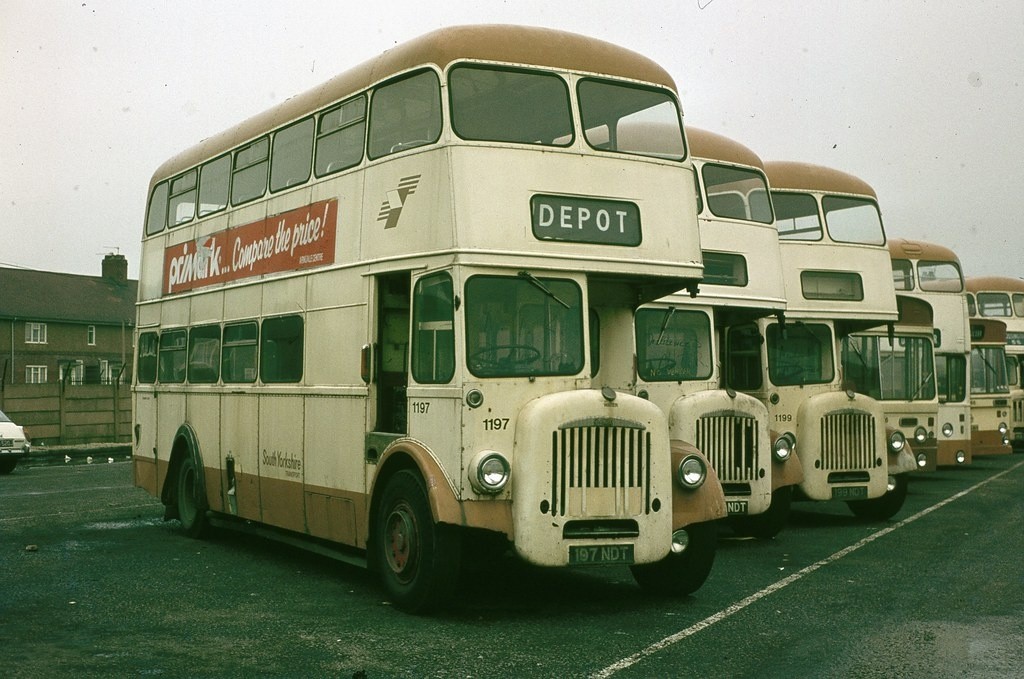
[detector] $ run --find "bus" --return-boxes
[534,119,805,539]
[732,159,918,540]
[921,275,1024,464]
[834,237,968,522]
[128,26,727,614]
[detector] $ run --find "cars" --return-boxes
[0,408,32,475]
